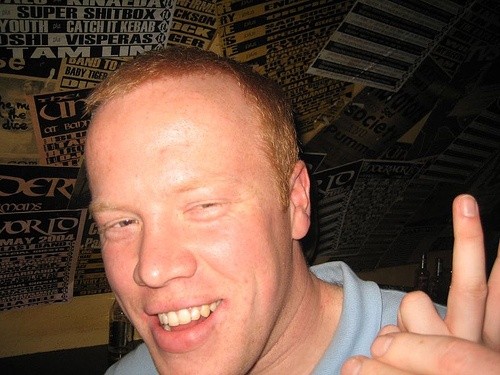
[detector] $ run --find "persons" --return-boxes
[83,43,499,373]
[375,192,500,351]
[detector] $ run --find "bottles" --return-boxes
[108,298,134,359]
[416,252,430,292]
[431,258,445,301]
